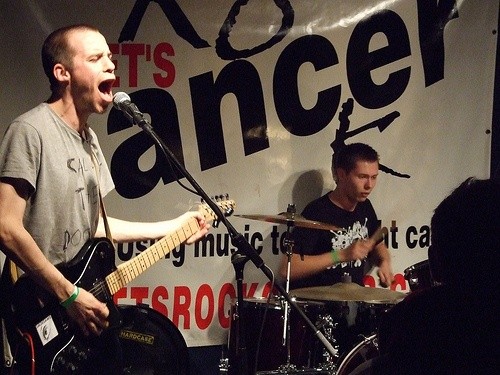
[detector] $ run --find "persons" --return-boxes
[280,142,395,329]
[0,24,211,375]
[374,176,500,375]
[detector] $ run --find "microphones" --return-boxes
[113,91,152,132]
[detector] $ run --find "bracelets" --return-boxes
[58,284,79,309]
[332,250,341,265]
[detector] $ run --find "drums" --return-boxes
[336,334,389,375]
[220,295,332,375]
[104,304,189,375]
[404,259,430,292]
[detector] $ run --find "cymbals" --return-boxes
[233,210,346,231]
[288,282,407,301]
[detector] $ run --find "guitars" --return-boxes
[11,194,236,375]
